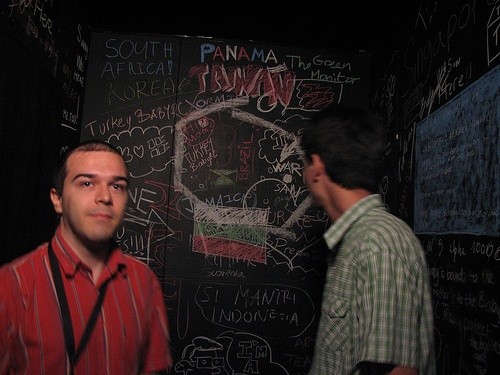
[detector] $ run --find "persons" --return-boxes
[0,140,174,375]
[300,105,438,375]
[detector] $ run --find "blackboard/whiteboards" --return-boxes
[0,1,500,375]
